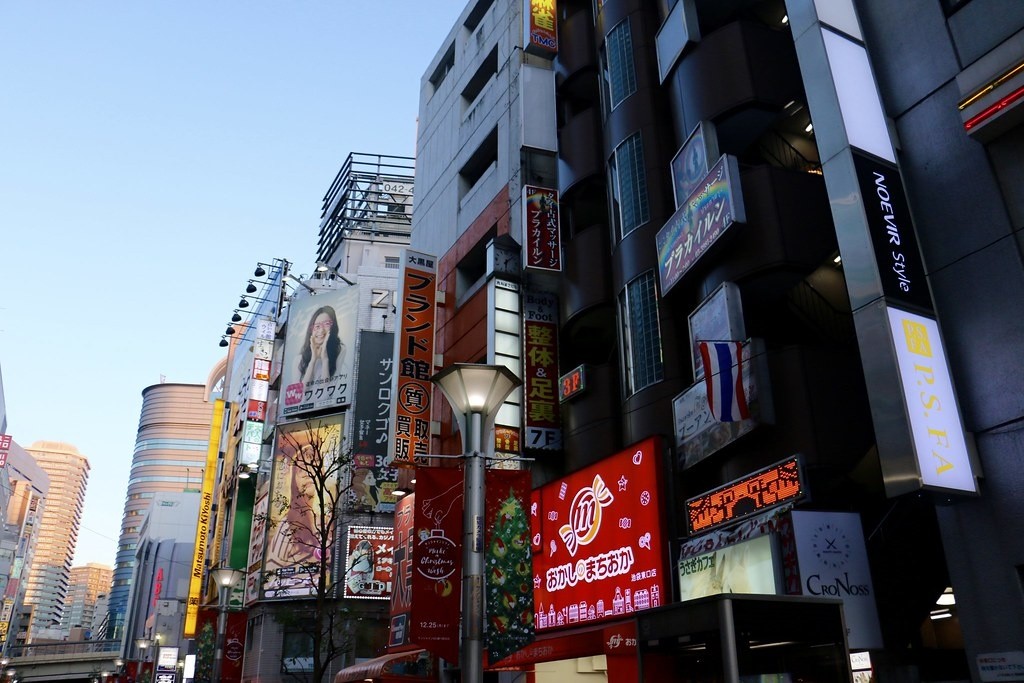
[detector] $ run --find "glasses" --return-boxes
[312,321,333,330]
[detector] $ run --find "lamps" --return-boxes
[246,279,280,293]
[282,274,314,292]
[219,335,253,347]
[254,262,282,276]
[317,261,356,286]
[239,462,269,490]
[225,322,256,335]
[239,294,277,308]
[391,488,412,495]
[232,309,270,323]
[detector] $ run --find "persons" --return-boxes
[298,305,347,399]
[264,444,333,591]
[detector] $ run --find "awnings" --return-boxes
[334,648,428,683]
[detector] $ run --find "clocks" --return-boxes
[486,233,522,274]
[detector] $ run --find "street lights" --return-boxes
[210,567,248,681]
[112,656,127,683]
[133,637,154,683]
[99,670,110,683]
[428,362,526,683]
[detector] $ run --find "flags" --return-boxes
[699,339,752,421]
